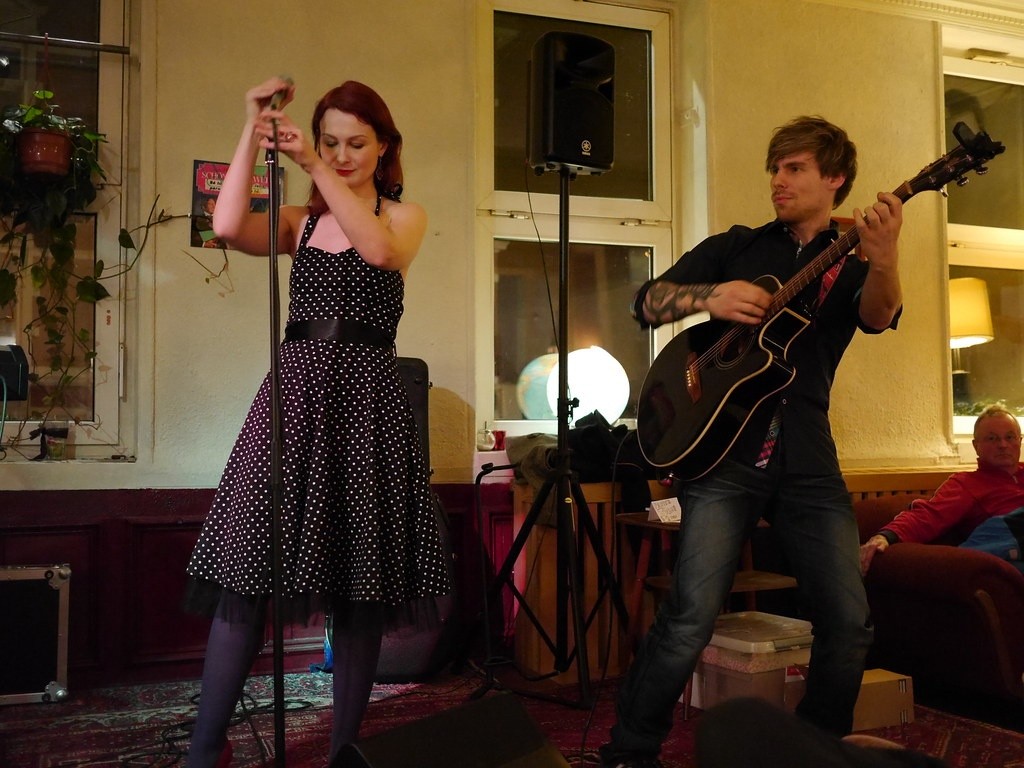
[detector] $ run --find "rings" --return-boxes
[285,132,294,143]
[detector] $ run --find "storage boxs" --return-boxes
[0,563,72,705]
[784,669,914,730]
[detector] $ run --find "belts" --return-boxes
[281,319,393,354]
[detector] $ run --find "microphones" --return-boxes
[271,75,294,112]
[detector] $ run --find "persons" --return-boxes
[860,405,1024,579]
[597,114,903,768]
[175,76,454,768]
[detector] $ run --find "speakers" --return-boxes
[320,691,569,768]
[528,31,615,177]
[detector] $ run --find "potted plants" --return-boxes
[0,90,233,462]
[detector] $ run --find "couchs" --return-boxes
[792,494,1024,732]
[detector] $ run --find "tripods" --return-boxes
[450,172,632,713]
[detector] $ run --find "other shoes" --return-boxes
[601,740,661,768]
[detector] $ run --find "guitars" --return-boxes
[637,121,1007,482]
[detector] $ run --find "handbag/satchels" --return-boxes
[567,411,636,480]
[505,433,587,488]
